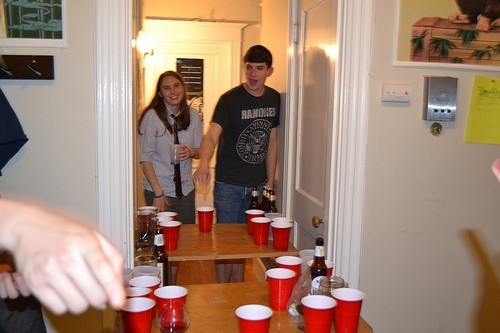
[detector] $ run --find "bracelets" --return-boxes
[153,191,164,198]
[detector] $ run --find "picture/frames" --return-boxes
[392,0,500,72]
[0,0,67,48]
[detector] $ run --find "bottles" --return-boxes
[249,186,277,213]
[310,238,328,279]
[151,227,169,285]
[318,277,345,298]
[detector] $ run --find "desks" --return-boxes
[111,224,374,333]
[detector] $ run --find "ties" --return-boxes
[171,114,182,199]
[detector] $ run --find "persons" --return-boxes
[191,45,281,285]
[138,70,203,285]
[0,88,128,317]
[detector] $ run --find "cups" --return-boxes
[274,256,303,286]
[120,298,155,333]
[169,144,182,164]
[156,302,190,333]
[134,255,157,267]
[246,209,294,251]
[133,266,161,279]
[134,205,182,252]
[307,259,334,277]
[128,276,162,293]
[299,248,315,276]
[264,267,296,312]
[123,268,133,287]
[196,207,215,232]
[330,288,365,333]
[125,286,153,299]
[300,295,336,333]
[234,304,274,333]
[154,285,188,326]
